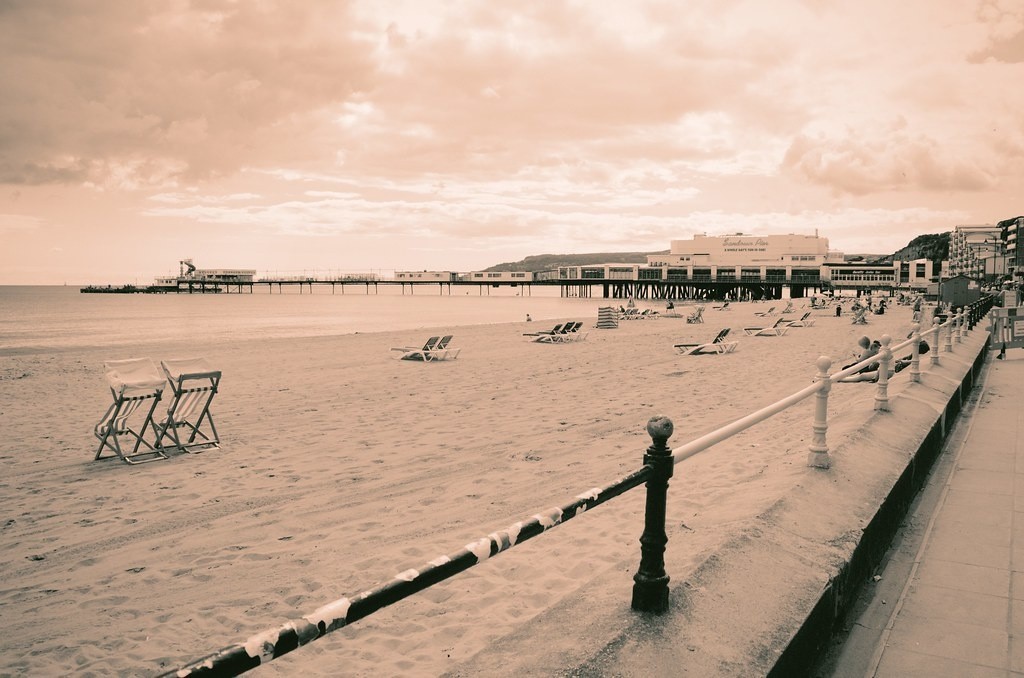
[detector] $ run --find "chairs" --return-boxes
[522,321,584,344]
[391,335,461,363]
[153,358,222,455]
[94,356,170,465]
[617,293,852,356]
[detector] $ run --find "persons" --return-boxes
[526,313,532,321]
[666,300,674,309]
[865,293,872,310]
[981,279,1024,325]
[842,336,874,373]
[910,296,920,313]
[619,304,625,313]
[836,305,842,317]
[895,331,930,372]
[719,300,729,311]
[811,294,817,306]
[837,340,895,381]
[899,293,904,302]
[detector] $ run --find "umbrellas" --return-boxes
[823,291,832,297]
